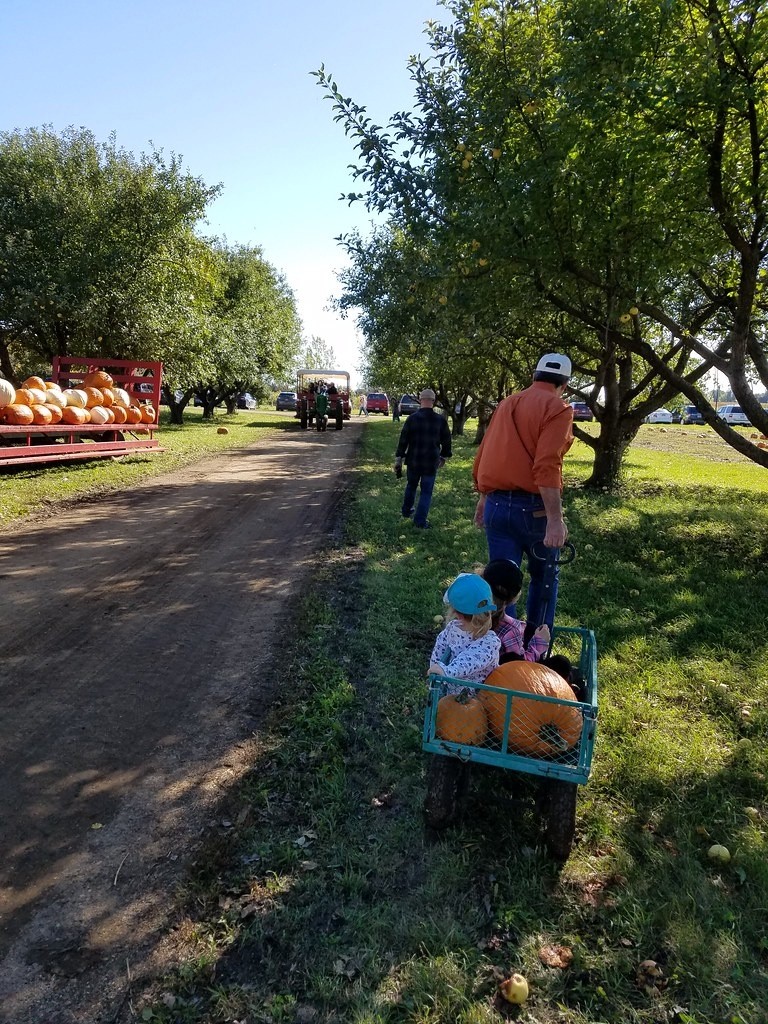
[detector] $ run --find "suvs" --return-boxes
[398,394,420,415]
[717,406,752,427]
[570,402,594,422]
[672,405,706,425]
[366,393,389,415]
[277,392,297,410]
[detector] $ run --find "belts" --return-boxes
[494,488,542,499]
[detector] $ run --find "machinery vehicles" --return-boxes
[294,369,351,432]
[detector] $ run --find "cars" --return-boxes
[644,409,673,423]
[158,389,256,409]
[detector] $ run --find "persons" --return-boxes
[391,398,400,422]
[472,351,572,635]
[476,557,572,685]
[395,389,453,530]
[307,380,330,395]
[326,383,338,394]
[358,394,369,417]
[426,572,501,697]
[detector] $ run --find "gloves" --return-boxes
[394,456,402,472]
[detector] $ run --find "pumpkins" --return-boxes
[0,371,156,425]
[217,427,227,434]
[436,660,583,758]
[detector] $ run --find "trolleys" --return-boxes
[422,539,598,859]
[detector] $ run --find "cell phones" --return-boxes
[396,466,402,479]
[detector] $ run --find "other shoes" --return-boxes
[425,519,429,528]
[411,506,416,514]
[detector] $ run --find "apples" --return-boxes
[400,483,765,721]
[739,738,752,747]
[501,973,528,1003]
[707,845,731,864]
[745,807,758,815]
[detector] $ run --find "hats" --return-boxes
[485,557,524,602]
[442,573,496,612]
[419,389,435,398]
[536,352,572,376]
